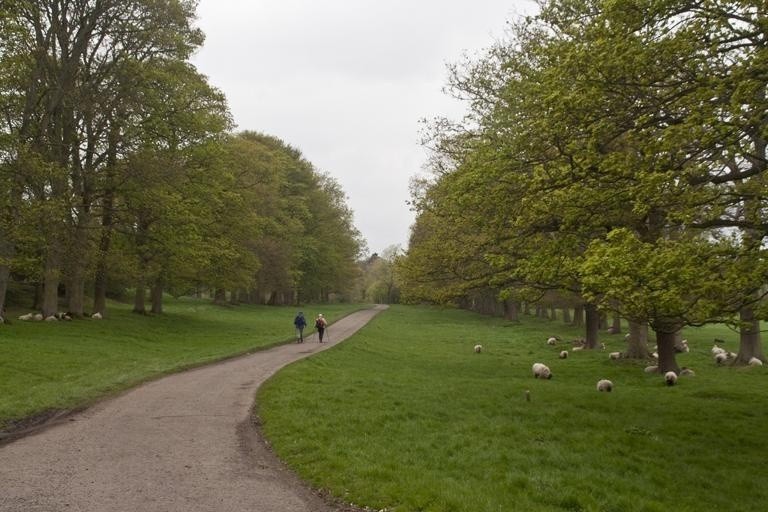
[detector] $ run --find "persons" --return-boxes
[313,314,327,343]
[294,312,307,344]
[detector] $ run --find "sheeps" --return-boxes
[547,338,557,345]
[474,345,482,353]
[748,356,763,368]
[679,367,695,377]
[609,351,625,360]
[596,380,614,393]
[559,351,568,359]
[17,311,74,322]
[644,366,659,374]
[681,339,689,353]
[664,371,678,387]
[532,363,553,379]
[91,312,103,320]
[606,327,618,334]
[711,344,738,363]
[571,344,587,351]
